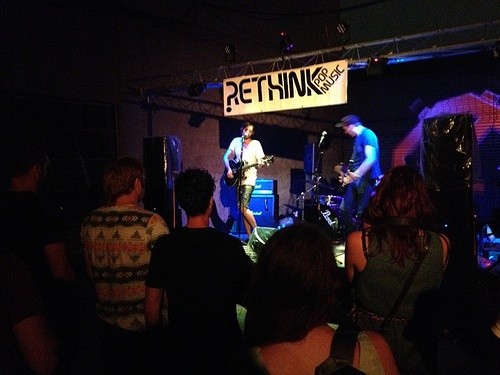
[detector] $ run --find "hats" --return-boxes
[335,114,359,128]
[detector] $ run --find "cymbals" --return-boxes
[305,179,334,190]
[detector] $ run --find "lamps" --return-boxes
[277,29,295,55]
[222,38,239,64]
[187,72,207,98]
[334,21,351,46]
[364,56,388,78]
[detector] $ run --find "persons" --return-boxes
[223,122,271,240]
[335,114,379,241]
[0,143,500,375]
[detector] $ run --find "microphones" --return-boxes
[242,134,248,138]
[318,131,327,147]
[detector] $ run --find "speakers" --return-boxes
[304,142,323,174]
[241,194,279,234]
[422,113,477,259]
[141,135,182,231]
[290,168,306,196]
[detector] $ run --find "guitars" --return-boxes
[224,155,274,186]
[334,163,345,178]
